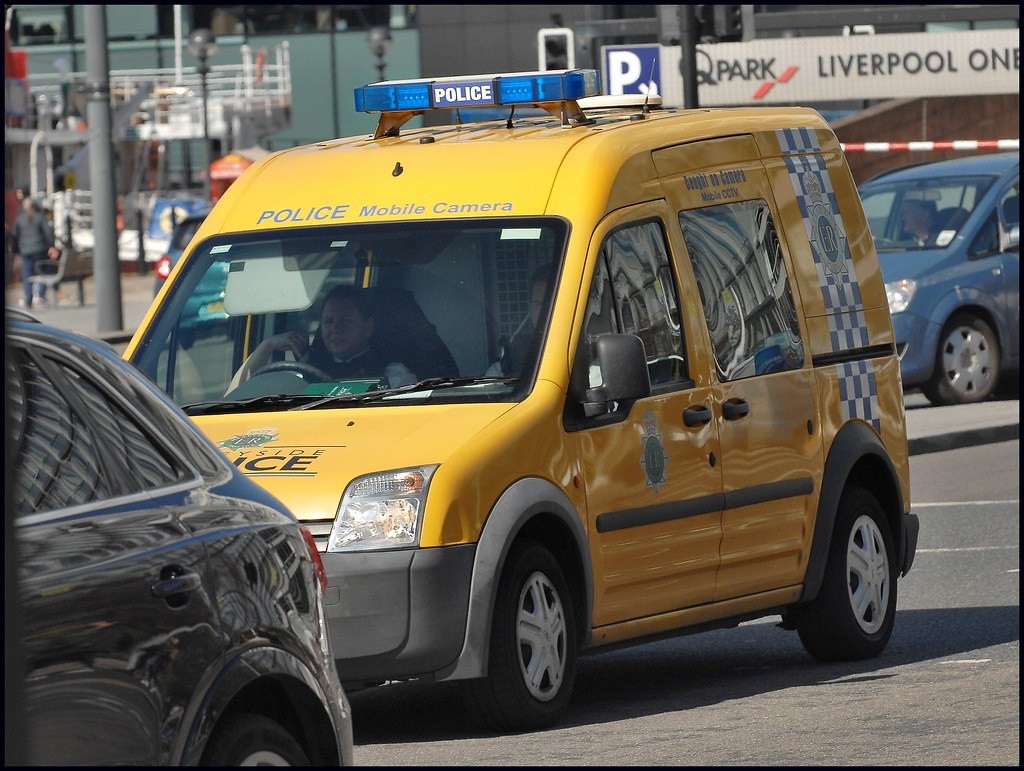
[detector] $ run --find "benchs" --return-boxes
[29,246,94,309]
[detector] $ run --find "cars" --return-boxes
[151,210,238,350]
[2,303,358,769]
[844,148,1022,406]
[31,182,214,284]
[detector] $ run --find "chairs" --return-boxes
[300,286,461,381]
[933,207,969,232]
[1003,196,1021,223]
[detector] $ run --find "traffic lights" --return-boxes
[537,24,575,71]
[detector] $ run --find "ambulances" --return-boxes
[109,69,923,736]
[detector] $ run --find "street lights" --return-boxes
[187,30,218,204]
[365,24,395,85]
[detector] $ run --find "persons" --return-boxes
[225,282,417,397]
[902,197,939,246]
[5,198,73,309]
[484,263,559,383]
